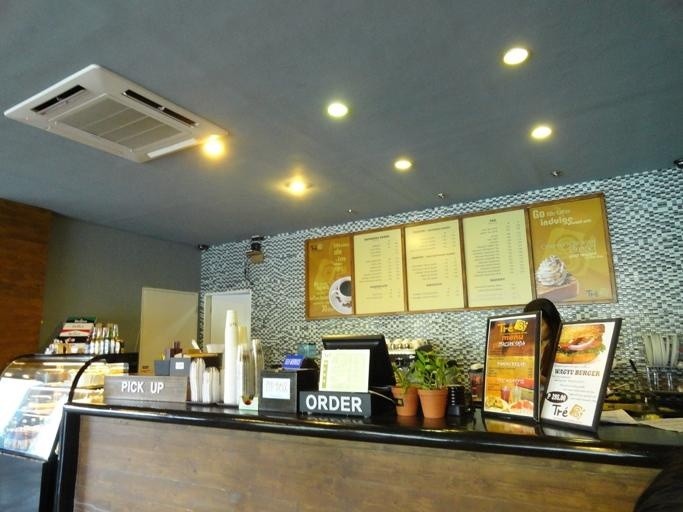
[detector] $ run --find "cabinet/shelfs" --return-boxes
[0,351,141,512]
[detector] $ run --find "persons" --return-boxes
[522,298,562,403]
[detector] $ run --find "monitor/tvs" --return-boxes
[322,335,396,387]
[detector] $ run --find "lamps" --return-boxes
[285,179,311,195]
[201,134,225,162]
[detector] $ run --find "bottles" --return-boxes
[632,372,644,391]
[88,322,123,356]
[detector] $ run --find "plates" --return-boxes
[328,276,352,315]
[484,395,509,412]
[507,400,534,417]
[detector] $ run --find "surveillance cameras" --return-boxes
[199,245,208,252]
[674,159,683,169]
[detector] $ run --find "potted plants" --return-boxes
[389,362,420,417]
[409,350,464,419]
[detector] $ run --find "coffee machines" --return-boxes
[388,345,433,368]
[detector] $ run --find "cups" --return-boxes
[511,387,522,404]
[647,365,679,393]
[448,384,465,406]
[331,279,352,304]
[499,383,510,403]
[387,337,428,350]
[189,309,264,409]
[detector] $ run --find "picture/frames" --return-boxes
[539,322,622,433]
[481,309,542,425]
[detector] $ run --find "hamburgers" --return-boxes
[555,324,606,364]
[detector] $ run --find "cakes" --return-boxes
[533,257,578,301]
